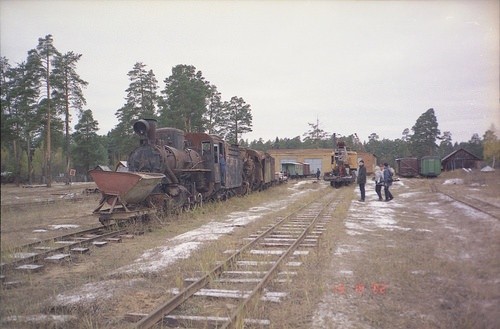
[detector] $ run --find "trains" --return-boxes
[281,161,310,178]
[323,141,359,189]
[89,117,276,227]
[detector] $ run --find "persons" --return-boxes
[372,162,395,202]
[357,160,366,202]
[315,168,321,180]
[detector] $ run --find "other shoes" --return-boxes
[378,198,383,201]
[358,199,364,202]
[389,197,393,200]
[385,199,390,202]
